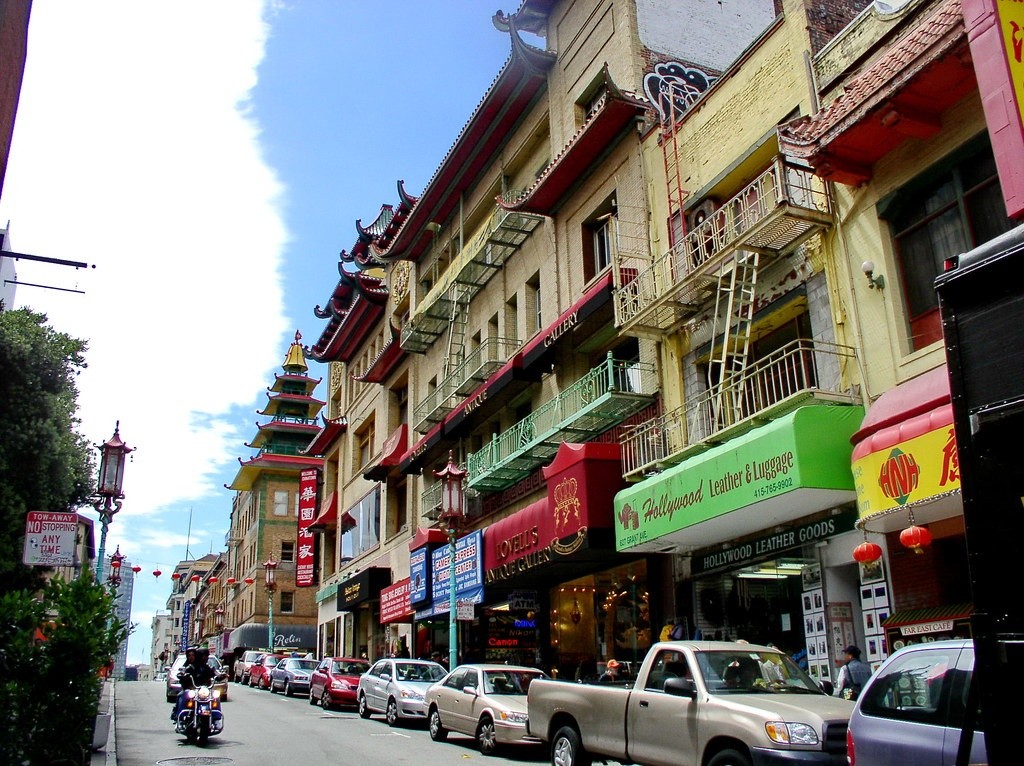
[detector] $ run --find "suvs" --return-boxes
[845,638,1023,766]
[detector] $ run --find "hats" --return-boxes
[842,645,861,657]
[607,659,621,668]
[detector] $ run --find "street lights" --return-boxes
[215,603,226,660]
[432,450,470,672]
[261,552,281,653]
[105,544,128,642]
[86,419,138,587]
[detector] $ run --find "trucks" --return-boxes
[935,204,1022,765]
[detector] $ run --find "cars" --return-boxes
[164,653,229,703]
[356,658,449,726]
[308,657,372,710]
[269,657,321,697]
[249,653,301,690]
[422,664,552,756]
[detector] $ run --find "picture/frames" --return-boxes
[802,563,823,592]
[859,555,884,585]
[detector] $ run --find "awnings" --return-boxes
[306,491,338,532]
[363,423,407,482]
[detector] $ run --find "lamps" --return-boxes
[861,261,885,290]
[736,561,809,579]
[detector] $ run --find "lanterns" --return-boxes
[173,573,181,580]
[900,525,932,554]
[209,576,218,584]
[111,560,120,569]
[153,568,162,579]
[852,541,882,570]
[132,565,142,575]
[228,577,235,585]
[245,577,254,584]
[191,575,200,583]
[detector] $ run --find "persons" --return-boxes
[722,640,787,686]
[430,651,450,674]
[836,645,872,699]
[172,646,229,729]
[291,650,314,660]
[575,655,625,682]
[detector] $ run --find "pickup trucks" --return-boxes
[234,650,270,684]
[522,640,857,766]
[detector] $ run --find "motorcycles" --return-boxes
[175,666,227,747]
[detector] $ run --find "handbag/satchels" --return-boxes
[843,683,861,701]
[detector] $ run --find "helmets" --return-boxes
[195,647,210,663]
[186,647,197,659]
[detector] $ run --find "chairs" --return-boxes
[655,661,693,689]
[715,666,760,694]
[455,676,471,691]
[405,668,419,679]
[492,676,512,694]
[924,677,980,714]
